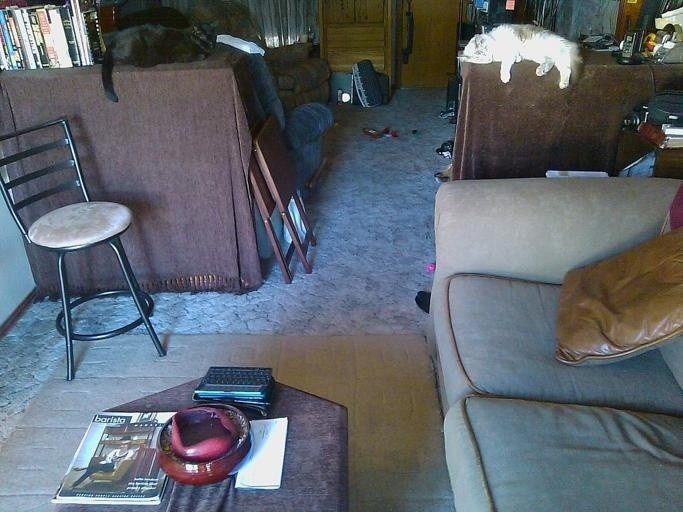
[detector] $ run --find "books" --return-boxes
[0,2,114,74]
[637,122,682,150]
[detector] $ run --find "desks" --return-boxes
[453,49,683,178]
[0,51,257,294]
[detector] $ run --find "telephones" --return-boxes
[617,32,638,65]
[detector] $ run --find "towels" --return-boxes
[216,34,265,56]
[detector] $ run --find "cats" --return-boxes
[455,21,584,91]
[100,16,220,103]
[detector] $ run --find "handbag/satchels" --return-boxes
[648,89,683,126]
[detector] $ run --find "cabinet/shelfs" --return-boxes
[449,0,558,124]
[613,128,683,179]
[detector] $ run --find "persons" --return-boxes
[71,435,135,488]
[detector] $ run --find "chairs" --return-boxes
[0,115,167,382]
[248,53,336,207]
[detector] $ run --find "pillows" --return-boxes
[554,226,683,366]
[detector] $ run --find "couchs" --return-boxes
[192,0,331,109]
[429,176,683,512]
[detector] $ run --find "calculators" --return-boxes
[194,366,273,400]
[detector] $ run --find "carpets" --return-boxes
[0,332,458,512]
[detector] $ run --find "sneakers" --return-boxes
[415,290,430,314]
